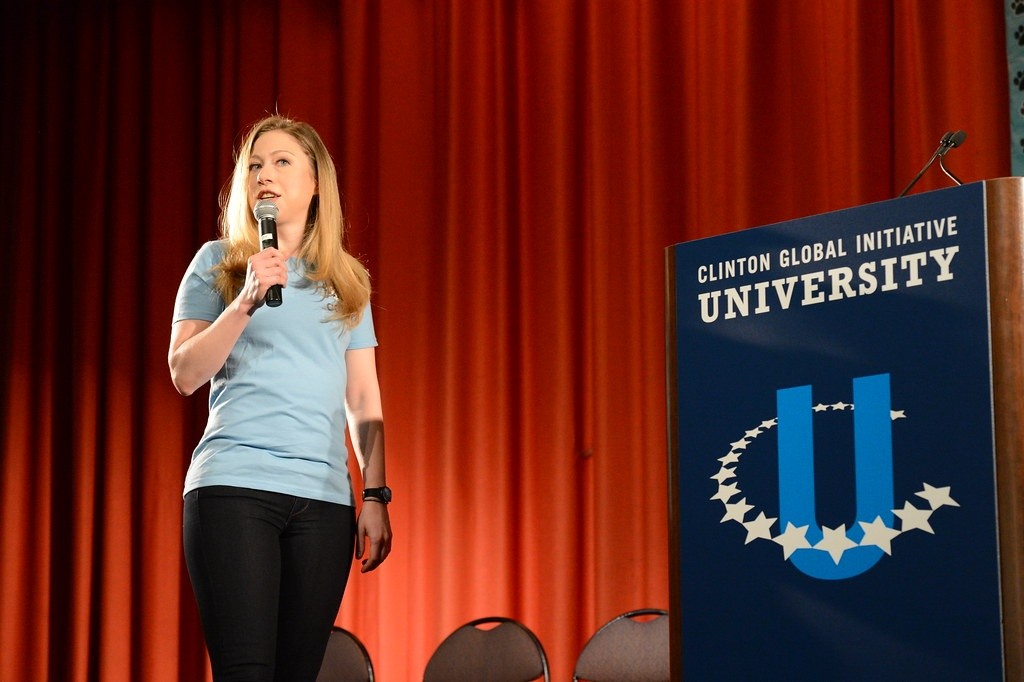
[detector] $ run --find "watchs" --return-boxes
[362,486,392,505]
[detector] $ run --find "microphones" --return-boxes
[253,200,283,307]
[896,130,966,198]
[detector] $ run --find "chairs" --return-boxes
[422,616,552,682]
[315,626,375,682]
[571,608,671,682]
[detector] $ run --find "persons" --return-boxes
[167,101,393,682]
